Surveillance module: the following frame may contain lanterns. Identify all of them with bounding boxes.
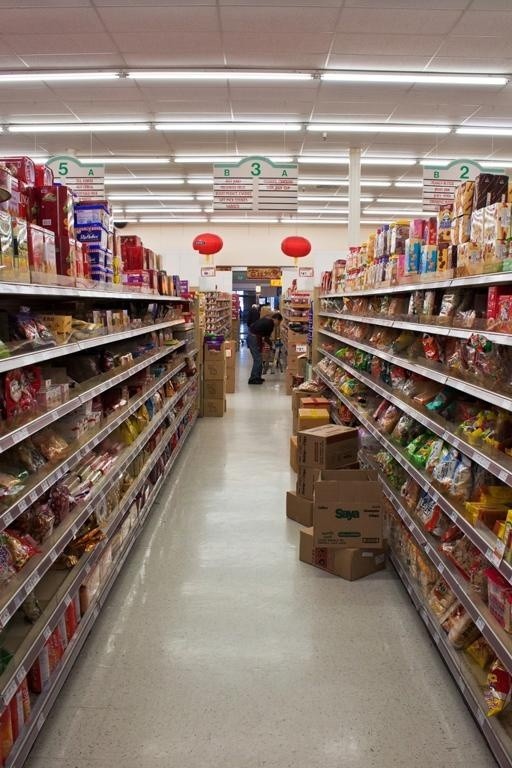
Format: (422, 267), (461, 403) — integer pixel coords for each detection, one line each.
(281, 236), (311, 271)
(192, 233), (223, 262)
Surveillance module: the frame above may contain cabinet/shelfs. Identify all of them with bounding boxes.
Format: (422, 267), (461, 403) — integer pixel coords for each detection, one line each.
(0, 283), (200, 768)
(317, 272), (512, 766)
(279, 297), (310, 375)
(199, 291), (240, 351)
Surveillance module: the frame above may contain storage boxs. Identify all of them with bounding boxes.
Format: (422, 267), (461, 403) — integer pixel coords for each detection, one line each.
(287, 328), (308, 396)
(203, 341), (235, 417)
(287, 387), (393, 581)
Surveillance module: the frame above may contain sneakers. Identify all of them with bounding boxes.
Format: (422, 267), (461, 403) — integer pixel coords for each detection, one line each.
(247, 377), (265, 385)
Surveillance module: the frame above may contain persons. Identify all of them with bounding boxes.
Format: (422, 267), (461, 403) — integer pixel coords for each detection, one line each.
(239, 304), (260, 329)
(247, 312), (283, 385)
